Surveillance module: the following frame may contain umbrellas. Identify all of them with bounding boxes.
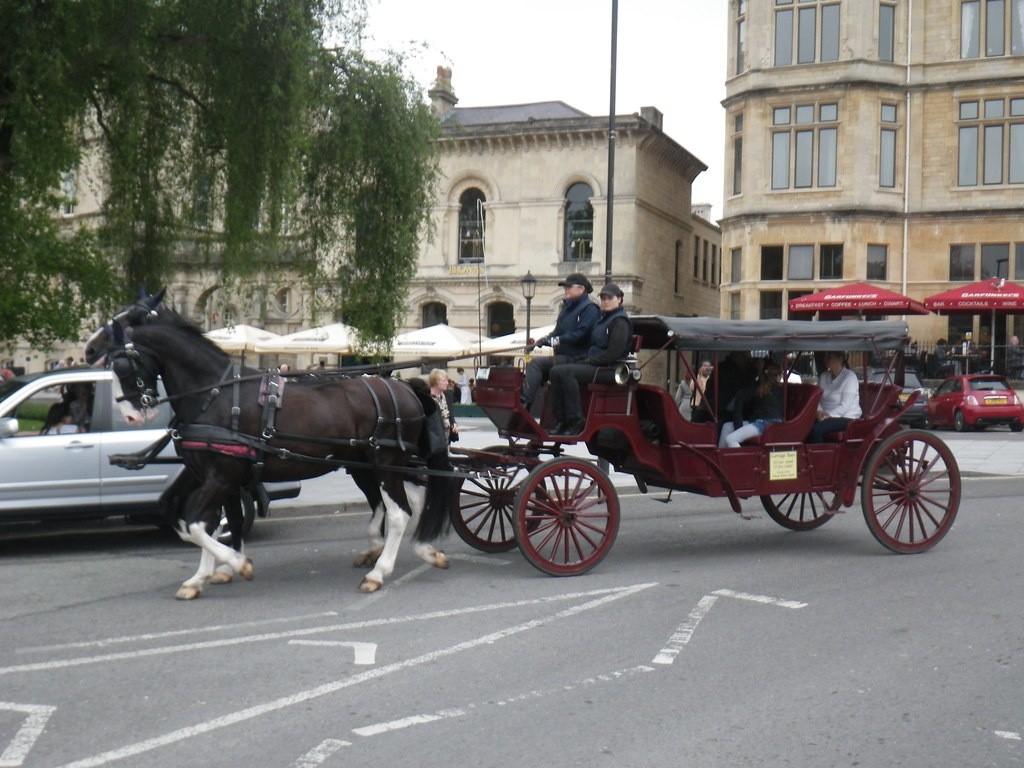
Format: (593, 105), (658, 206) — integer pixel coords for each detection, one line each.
(924, 275), (1024, 376)
(199, 320), (558, 367)
(791, 284), (929, 320)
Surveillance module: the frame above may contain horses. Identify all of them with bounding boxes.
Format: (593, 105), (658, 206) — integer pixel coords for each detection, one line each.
(86, 286), (449, 599)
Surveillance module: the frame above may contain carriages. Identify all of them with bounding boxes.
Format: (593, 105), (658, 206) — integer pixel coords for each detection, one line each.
(83, 284), (962, 600)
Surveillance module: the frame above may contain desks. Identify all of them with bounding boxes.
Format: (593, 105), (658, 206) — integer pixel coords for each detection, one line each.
(951, 354), (978, 375)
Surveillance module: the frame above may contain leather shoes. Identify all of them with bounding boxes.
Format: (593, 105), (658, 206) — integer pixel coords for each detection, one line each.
(549, 417), (587, 436)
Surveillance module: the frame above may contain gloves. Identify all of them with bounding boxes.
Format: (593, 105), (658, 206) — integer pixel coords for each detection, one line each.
(536, 337), (551, 348)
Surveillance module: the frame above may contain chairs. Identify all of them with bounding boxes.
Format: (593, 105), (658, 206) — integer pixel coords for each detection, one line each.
(921, 350), (929, 364)
(926, 354), (948, 379)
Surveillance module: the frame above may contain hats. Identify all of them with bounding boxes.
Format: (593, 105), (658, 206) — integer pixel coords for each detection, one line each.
(597, 284), (624, 298)
(558, 273), (593, 293)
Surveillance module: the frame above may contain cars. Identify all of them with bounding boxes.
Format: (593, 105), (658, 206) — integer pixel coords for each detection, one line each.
(0, 366), (302, 546)
(923, 374), (1024, 433)
(847, 365), (929, 428)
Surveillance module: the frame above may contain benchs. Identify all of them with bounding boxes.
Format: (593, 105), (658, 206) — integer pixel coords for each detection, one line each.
(825, 382), (903, 442)
(637, 384), (717, 447)
(746, 380), (825, 446)
(585, 334), (644, 415)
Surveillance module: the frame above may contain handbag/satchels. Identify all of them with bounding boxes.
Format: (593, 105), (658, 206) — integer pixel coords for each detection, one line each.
(449, 429), (459, 442)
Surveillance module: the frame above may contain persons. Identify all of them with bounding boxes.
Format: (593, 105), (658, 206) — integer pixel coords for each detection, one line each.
(457, 367), (472, 404)
(447, 380), (456, 389)
(690, 349), (862, 450)
(53, 357), (73, 370)
(469, 379), (474, 392)
(520, 273), (600, 413)
(675, 365), (695, 422)
(549, 284), (633, 436)
(428, 368), (459, 444)
(905, 336), (1024, 380)
(691, 361), (713, 422)
(41, 403), (85, 434)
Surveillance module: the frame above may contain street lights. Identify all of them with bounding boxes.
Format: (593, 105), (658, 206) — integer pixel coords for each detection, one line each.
(521, 270), (538, 371)
